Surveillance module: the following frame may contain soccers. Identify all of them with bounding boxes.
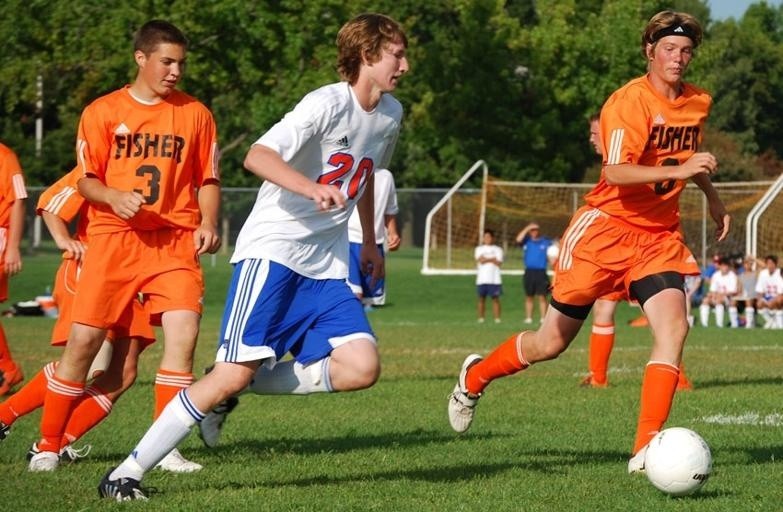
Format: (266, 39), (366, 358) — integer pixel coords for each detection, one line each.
(645, 427), (713, 500)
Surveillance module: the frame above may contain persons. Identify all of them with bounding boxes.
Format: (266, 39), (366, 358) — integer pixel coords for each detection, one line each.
(26, 20), (225, 474)
(1, 165), (158, 465)
(349, 169), (404, 307)
(474, 229), (503, 324)
(517, 223), (553, 323)
(97, 14), (410, 503)
(685, 251), (782, 329)
(447, 12), (734, 476)
(580, 114), (696, 392)
(1, 143), (30, 398)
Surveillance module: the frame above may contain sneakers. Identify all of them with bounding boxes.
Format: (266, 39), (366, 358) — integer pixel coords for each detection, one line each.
(98, 466), (156, 503)
(448, 355), (483, 433)
(154, 448), (203, 474)
(0, 364), (91, 473)
(628, 444), (648, 475)
(199, 363), (239, 450)
